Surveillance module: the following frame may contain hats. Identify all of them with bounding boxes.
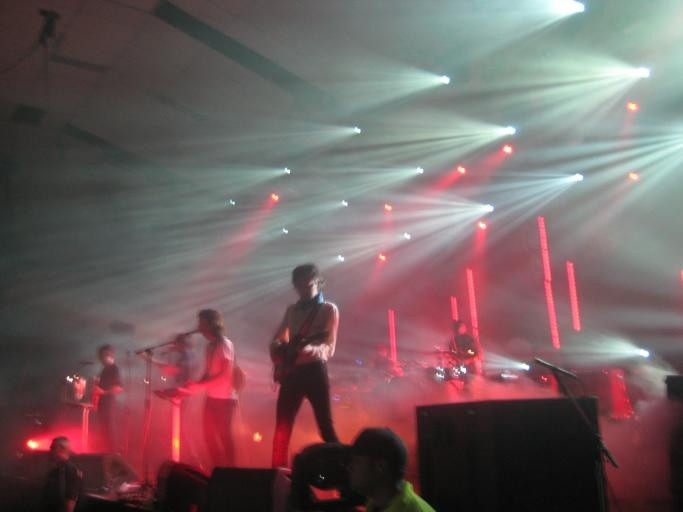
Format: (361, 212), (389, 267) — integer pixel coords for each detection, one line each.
(340, 426), (407, 466)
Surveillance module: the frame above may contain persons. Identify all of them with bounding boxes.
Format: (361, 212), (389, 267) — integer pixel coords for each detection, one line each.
(186, 307), (241, 466)
(448, 320), (481, 379)
(39, 437), (81, 512)
(269, 263), (340, 464)
(345, 426), (437, 512)
(139, 336), (201, 467)
(91, 345), (126, 456)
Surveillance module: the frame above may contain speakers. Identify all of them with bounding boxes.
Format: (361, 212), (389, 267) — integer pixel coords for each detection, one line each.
(152, 460), (208, 512)
(196, 467), (292, 512)
(69, 452), (138, 494)
(416, 396), (608, 512)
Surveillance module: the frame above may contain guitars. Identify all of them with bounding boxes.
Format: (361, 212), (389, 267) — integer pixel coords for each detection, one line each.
(151, 363), (246, 399)
(274, 329), (328, 383)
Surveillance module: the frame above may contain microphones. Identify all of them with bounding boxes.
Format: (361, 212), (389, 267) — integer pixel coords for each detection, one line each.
(534, 356), (579, 381)
(80, 362), (94, 364)
(191, 329), (199, 333)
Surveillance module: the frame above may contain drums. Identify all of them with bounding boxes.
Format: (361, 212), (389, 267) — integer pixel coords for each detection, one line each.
(447, 364), (468, 380)
(429, 364), (447, 382)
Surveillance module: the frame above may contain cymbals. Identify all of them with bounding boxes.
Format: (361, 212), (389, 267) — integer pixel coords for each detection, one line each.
(441, 350), (467, 360)
